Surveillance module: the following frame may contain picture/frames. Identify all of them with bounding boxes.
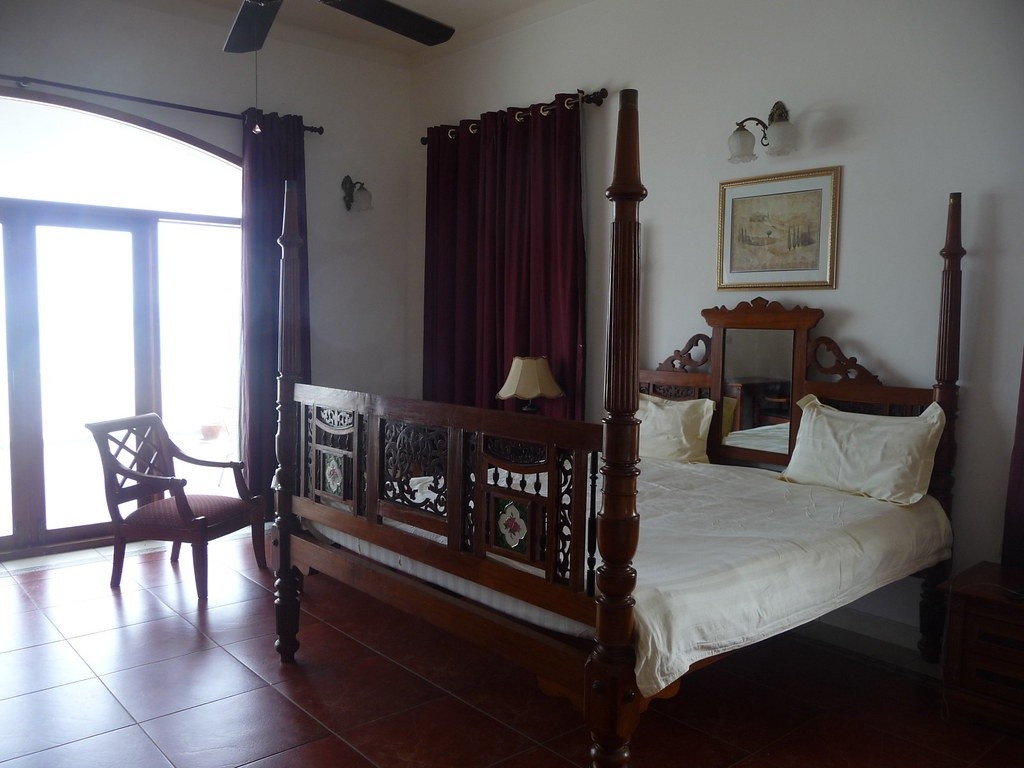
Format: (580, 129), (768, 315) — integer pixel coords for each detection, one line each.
(712, 164), (848, 292)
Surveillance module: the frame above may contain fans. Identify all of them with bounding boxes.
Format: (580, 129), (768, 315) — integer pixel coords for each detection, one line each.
(219, 0), (455, 59)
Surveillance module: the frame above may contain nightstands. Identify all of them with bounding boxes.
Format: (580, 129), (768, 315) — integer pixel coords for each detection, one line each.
(943, 559), (1023, 735)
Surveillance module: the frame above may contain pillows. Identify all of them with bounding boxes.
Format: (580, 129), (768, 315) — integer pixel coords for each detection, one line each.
(781, 392), (948, 508)
(632, 394), (715, 462)
(722, 397), (738, 443)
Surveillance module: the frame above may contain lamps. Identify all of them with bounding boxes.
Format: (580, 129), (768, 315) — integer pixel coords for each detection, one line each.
(496, 356), (565, 459)
(726, 101), (794, 166)
(337, 171), (376, 218)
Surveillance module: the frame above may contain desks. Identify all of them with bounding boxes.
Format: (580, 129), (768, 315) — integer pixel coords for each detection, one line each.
(724, 377), (790, 432)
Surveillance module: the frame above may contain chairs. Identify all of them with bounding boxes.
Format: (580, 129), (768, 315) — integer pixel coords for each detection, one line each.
(752, 394), (790, 428)
(84, 409), (268, 604)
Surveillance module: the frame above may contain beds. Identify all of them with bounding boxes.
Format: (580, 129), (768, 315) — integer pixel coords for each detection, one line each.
(271, 86), (968, 768)
(723, 422), (789, 455)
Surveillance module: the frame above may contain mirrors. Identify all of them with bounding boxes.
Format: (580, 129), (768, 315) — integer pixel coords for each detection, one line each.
(716, 321), (797, 465)
